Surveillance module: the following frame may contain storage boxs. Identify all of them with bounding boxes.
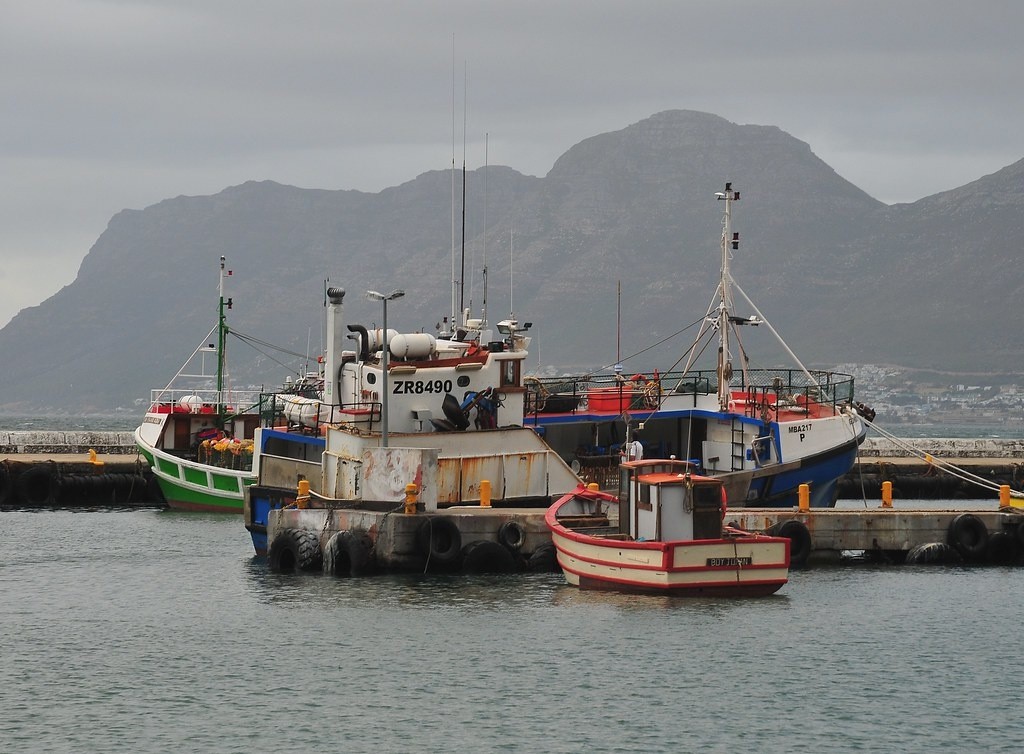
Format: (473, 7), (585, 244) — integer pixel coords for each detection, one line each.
(588, 387), (633, 411)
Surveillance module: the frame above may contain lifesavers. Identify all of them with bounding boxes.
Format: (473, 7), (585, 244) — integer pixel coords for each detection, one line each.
(947, 514), (990, 560)
(418, 516), (463, 560)
(498, 520), (526, 550)
(647, 382), (665, 395)
(772, 519), (813, 564)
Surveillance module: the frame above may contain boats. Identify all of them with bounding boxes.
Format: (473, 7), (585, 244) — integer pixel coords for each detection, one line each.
(523, 179), (875, 508)
(545, 414), (792, 593)
(134, 253), (325, 517)
(244, 289), (585, 579)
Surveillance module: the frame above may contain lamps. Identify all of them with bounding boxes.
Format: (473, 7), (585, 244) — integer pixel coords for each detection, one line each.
(455, 362), (483, 373)
(389, 365), (416, 375)
